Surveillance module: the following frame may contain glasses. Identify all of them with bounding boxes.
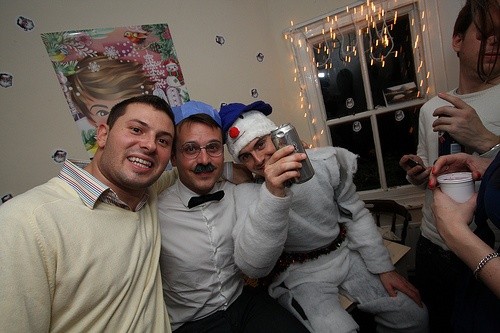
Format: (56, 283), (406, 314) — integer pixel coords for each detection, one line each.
(175, 142), (224, 159)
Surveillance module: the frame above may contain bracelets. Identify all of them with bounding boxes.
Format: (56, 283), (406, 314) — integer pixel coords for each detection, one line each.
(473, 253), (498, 274)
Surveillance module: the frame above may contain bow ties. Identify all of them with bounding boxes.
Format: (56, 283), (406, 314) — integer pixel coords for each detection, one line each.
(188, 190), (226, 209)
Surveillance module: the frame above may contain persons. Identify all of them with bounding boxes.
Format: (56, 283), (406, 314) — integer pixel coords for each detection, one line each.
(220, 101), (428, 333)
(399, 0), (500, 333)
(0, 95), (310, 333)
(156, 101), (311, 333)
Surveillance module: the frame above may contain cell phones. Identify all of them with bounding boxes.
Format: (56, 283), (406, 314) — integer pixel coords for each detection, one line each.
(407, 158), (426, 175)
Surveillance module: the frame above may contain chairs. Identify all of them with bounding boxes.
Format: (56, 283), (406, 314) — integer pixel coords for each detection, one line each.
(337, 240), (412, 309)
(361, 199), (412, 246)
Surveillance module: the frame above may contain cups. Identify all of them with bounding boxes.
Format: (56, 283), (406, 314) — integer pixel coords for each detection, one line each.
(438, 171), (476, 203)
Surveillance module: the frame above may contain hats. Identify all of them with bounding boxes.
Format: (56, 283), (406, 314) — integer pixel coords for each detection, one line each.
(218, 99), (278, 163)
(172, 100), (222, 129)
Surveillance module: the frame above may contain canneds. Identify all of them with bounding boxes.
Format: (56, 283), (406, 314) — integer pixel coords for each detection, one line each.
(438, 106), (465, 157)
(270, 123), (313, 183)
